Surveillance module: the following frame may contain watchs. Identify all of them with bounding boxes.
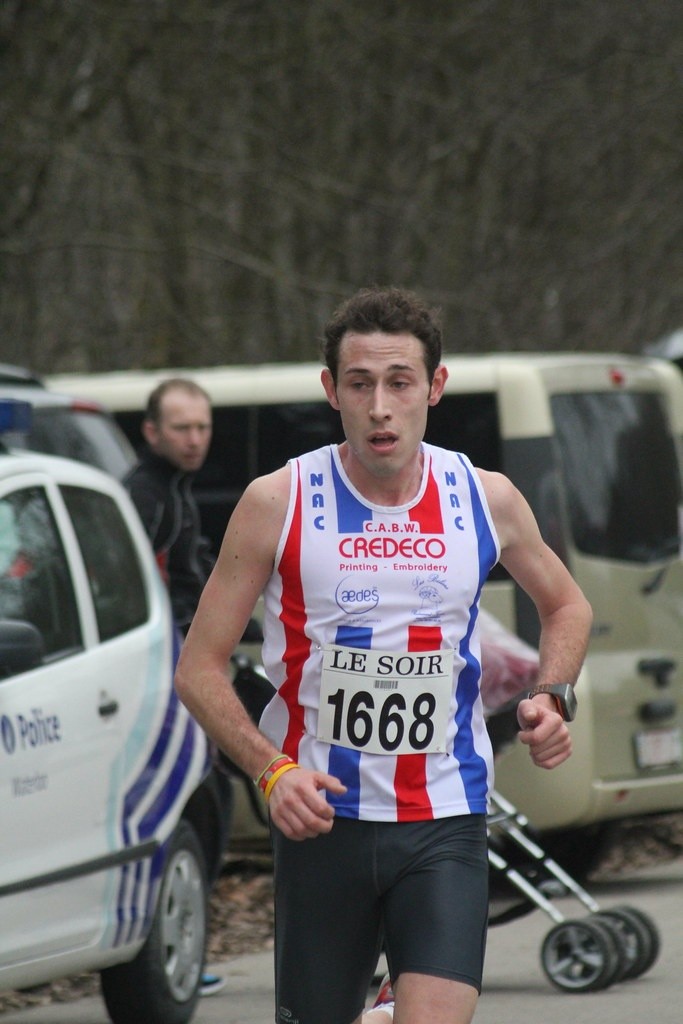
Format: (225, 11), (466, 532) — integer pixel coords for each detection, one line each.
(526, 683), (578, 722)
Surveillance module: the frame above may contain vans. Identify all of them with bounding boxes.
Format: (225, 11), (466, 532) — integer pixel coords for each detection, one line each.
(0, 353), (683, 894)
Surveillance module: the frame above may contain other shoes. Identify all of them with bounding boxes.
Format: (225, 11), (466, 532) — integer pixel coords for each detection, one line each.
(372, 973), (396, 1010)
(196, 976), (226, 996)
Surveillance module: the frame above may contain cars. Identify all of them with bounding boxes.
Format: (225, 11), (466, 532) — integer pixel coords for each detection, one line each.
(0, 453), (230, 1023)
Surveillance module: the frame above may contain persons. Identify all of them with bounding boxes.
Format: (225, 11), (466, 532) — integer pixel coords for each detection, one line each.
(120, 379), (276, 999)
(171, 287), (595, 1024)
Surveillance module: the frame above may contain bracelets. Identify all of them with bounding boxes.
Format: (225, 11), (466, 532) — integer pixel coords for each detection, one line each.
(254, 755), (301, 803)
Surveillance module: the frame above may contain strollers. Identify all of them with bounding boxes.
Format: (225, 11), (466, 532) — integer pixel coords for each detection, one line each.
(221, 621), (664, 996)
